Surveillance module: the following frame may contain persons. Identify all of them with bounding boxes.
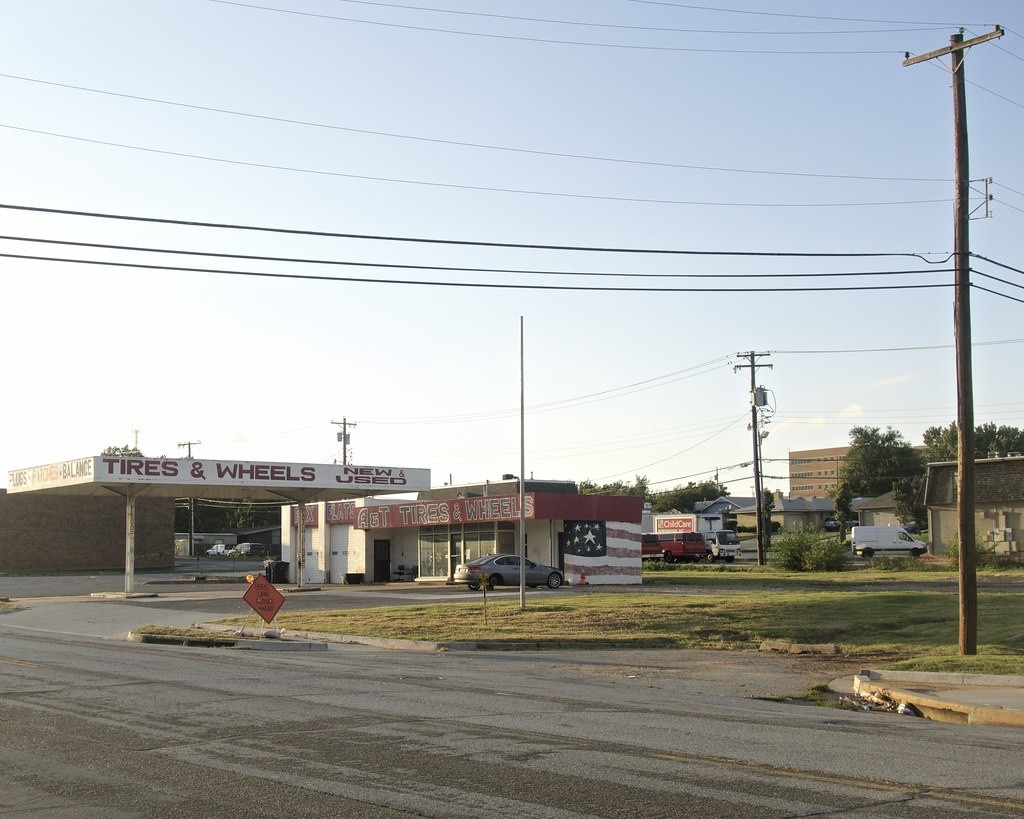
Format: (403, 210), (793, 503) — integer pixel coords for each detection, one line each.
(205, 546), (209, 558)
(897, 516), (905, 526)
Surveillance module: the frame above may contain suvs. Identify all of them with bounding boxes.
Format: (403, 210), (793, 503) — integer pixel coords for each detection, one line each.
(824, 516), (859, 532)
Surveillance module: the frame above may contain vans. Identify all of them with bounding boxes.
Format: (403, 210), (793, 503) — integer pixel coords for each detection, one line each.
(235, 543), (266, 556)
(850, 525), (928, 557)
(652, 533), (706, 563)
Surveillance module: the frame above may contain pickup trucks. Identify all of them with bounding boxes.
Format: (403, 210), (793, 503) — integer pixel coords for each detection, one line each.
(206, 543), (234, 558)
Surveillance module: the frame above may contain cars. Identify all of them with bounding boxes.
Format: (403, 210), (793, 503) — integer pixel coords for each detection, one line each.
(454, 552), (564, 591)
(903, 525), (921, 534)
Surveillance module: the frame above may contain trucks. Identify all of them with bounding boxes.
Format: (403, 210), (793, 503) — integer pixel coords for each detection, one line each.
(652, 514), (742, 563)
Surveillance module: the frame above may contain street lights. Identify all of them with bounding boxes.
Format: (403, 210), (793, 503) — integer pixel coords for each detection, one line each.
(757, 430), (770, 565)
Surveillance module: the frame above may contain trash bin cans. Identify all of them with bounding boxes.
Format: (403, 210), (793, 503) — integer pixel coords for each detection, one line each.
(264, 561), (271, 583)
(271, 561), (290, 583)
